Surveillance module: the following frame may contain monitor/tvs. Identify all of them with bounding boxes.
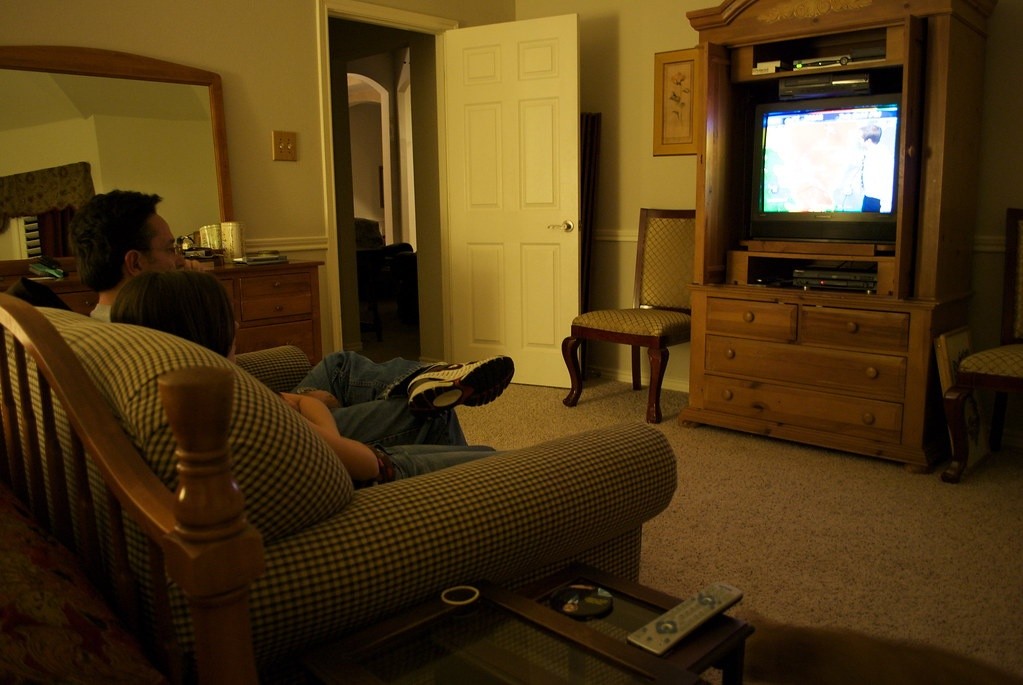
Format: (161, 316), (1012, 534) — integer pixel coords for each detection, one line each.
(749, 93), (903, 241)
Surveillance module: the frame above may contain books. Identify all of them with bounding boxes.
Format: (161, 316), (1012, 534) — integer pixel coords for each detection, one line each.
(233, 255), (289, 265)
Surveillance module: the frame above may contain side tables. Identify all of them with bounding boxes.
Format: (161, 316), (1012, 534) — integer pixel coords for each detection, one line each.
(301, 559), (755, 685)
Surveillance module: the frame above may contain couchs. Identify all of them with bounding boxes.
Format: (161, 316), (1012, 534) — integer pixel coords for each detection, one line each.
(0, 294), (679, 685)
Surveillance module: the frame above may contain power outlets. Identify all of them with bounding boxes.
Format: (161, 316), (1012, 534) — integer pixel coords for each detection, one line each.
(274, 130), (298, 162)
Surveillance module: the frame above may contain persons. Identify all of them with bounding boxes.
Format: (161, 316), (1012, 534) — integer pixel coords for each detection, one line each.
(859, 124), (881, 190)
(111, 271), (506, 485)
(68, 190), (514, 443)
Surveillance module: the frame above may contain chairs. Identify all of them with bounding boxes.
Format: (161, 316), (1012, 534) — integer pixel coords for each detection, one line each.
(562, 208), (695, 424)
(940, 205), (1023, 484)
(352, 218), (412, 342)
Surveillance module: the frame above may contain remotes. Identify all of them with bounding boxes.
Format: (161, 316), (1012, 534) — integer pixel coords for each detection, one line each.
(626, 582), (747, 657)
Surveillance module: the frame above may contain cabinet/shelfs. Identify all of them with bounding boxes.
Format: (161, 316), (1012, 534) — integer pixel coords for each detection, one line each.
(1, 255), (320, 387)
(678, 0), (998, 473)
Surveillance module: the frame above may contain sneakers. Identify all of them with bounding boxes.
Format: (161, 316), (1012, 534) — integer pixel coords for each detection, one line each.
(406, 355), (515, 417)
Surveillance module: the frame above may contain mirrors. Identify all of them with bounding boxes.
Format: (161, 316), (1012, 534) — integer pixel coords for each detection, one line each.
(0, 45), (233, 262)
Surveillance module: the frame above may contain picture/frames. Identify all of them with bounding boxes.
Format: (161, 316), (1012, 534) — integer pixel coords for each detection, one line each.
(652, 47), (700, 156)
(932, 326), (989, 469)
